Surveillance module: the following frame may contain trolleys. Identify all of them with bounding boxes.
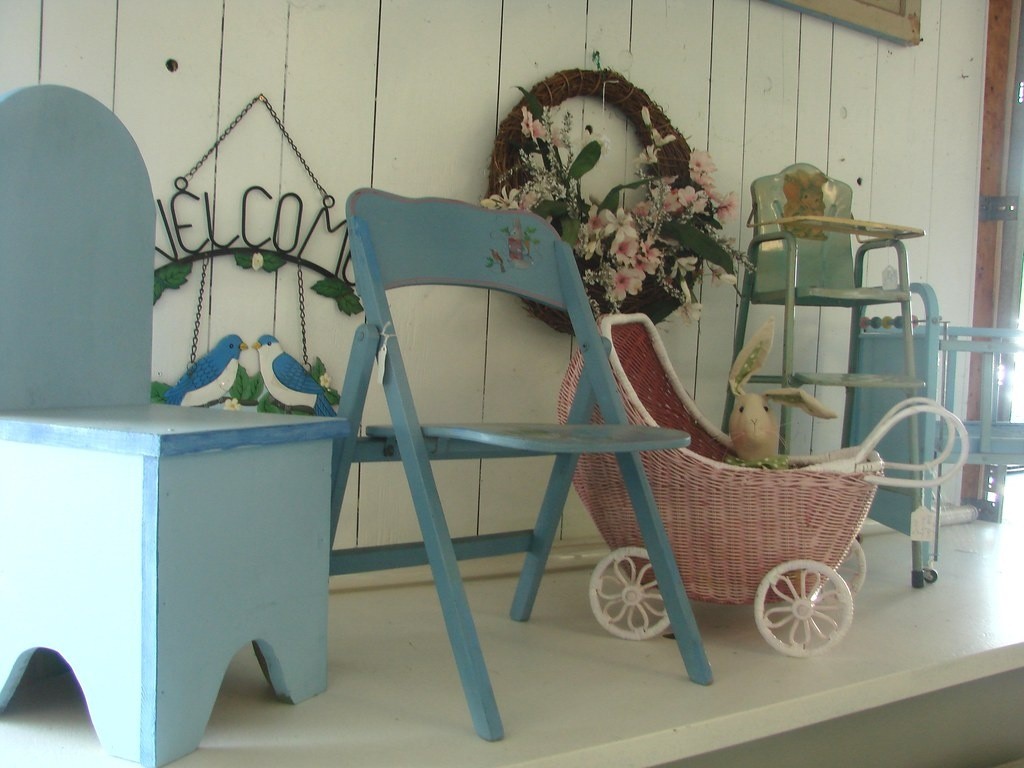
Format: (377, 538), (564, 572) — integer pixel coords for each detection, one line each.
(560, 316), (970, 658)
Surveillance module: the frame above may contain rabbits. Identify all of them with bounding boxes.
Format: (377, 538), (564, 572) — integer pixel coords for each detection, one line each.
(721, 317), (839, 470)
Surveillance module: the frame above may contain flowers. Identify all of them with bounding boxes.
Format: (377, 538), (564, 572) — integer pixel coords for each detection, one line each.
(481, 61), (753, 328)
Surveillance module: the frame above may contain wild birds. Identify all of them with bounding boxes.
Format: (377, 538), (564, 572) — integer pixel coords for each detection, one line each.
(162, 333), (251, 408)
(252, 333), (338, 420)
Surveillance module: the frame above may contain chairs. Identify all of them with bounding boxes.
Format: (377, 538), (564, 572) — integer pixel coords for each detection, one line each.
(847, 275), (1023, 581)
(1, 84), (340, 766)
(720, 164), (938, 584)
(298, 184), (716, 740)
